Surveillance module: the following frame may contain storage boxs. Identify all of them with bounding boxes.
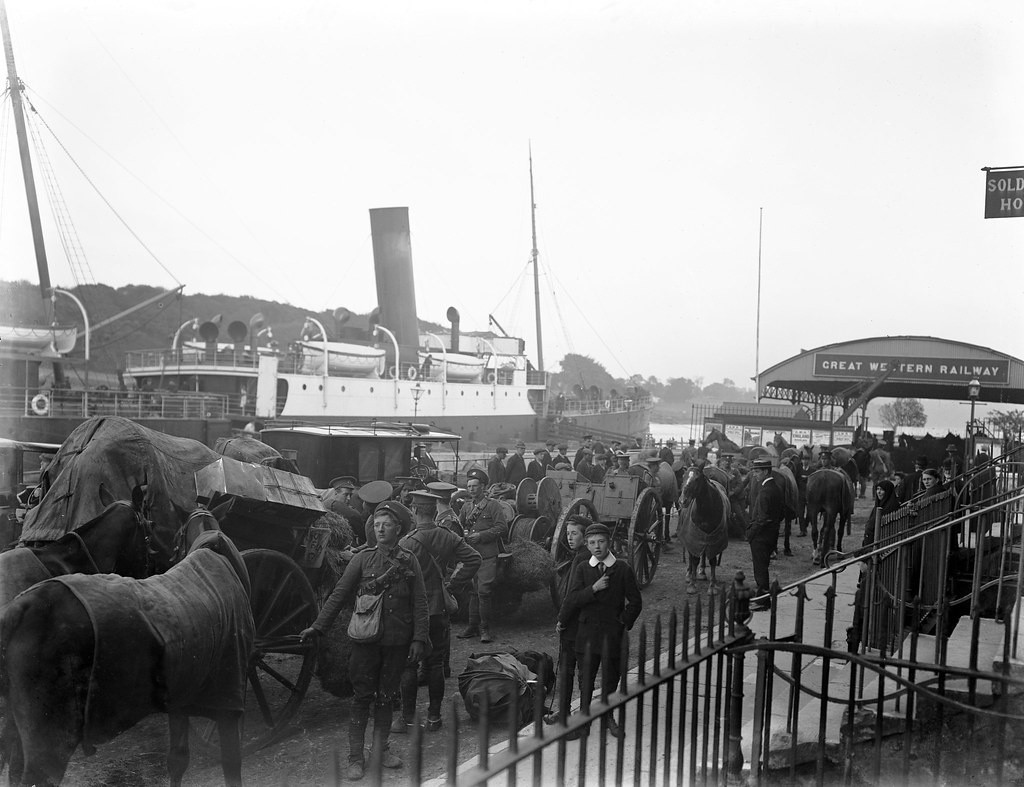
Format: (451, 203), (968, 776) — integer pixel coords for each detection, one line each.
(546, 470), (651, 521)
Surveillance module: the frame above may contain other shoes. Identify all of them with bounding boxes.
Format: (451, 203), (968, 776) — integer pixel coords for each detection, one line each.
(797, 531), (806, 537)
(750, 599), (772, 611)
(347, 755), (365, 780)
(390, 717), (412, 732)
(369, 745), (403, 768)
(480, 624), (492, 641)
(426, 715), (443, 731)
(609, 718), (624, 738)
(456, 625), (480, 638)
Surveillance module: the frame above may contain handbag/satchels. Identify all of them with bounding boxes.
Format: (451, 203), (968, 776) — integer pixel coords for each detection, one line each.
(346, 594), (384, 643)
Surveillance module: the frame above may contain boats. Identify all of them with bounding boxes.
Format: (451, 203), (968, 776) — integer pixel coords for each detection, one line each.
(1, 209), (652, 458)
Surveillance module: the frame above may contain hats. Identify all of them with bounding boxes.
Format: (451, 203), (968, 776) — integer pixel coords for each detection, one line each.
(819, 451), (832, 458)
(584, 524), (610, 534)
(358, 481), (393, 504)
(375, 500), (411, 536)
(329, 476), (357, 489)
(749, 459), (776, 469)
(394, 476), (421, 486)
(721, 455), (734, 461)
(912, 454), (928, 466)
(569, 515), (592, 525)
(495, 435), (630, 469)
(946, 444), (957, 451)
(409, 491), (443, 504)
(427, 482), (457, 495)
(467, 469), (489, 484)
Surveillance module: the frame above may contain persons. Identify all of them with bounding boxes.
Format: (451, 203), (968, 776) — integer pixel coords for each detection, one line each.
(57, 378), (248, 418)
(563, 524), (641, 740)
(527, 361), (535, 385)
(391, 490), (483, 733)
(21, 452), (54, 510)
(299, 501), (429, 780)
(421, 355), (433, 382)
(329, 436), (996, 637)
(544, 514), (604, 723)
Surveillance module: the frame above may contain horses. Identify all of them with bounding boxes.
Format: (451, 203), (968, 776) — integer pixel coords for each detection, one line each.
(701, 424), (897, 569)
(0, 482), (155, 609)
(0, 496), (256, 787)
(676, 462), (731, 596)
